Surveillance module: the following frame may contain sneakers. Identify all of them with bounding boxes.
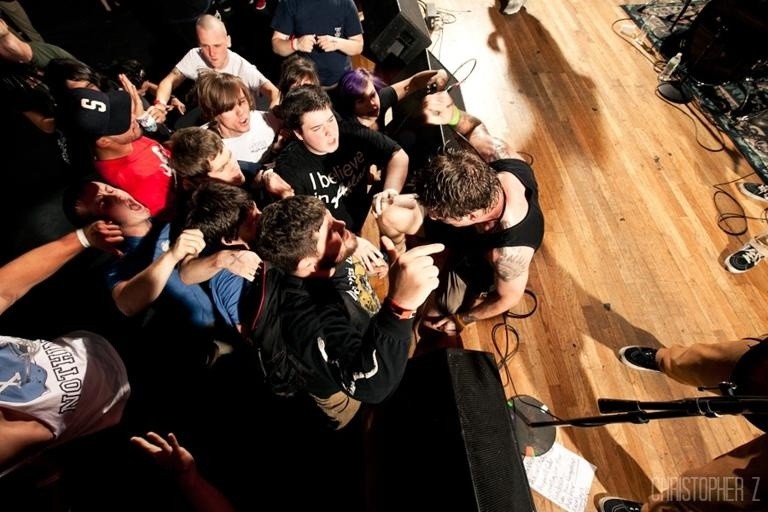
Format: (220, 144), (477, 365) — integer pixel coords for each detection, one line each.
(599, 494), (643, 512)
(739, 181), (767, 201)
(618, 344), (661, 373)
(724, 240), (761, 274)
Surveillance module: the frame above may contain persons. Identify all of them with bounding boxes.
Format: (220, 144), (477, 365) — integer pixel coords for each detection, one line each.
(723, 179), (768, 276)
(593, 332), (768, 511)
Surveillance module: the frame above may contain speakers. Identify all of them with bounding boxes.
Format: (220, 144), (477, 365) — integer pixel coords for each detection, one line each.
(361, 0), (432, 72)
(391, 49), (471, 181)
(380, 347), (535, 512)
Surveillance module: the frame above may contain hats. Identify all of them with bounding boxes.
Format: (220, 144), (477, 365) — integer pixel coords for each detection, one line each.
(54, 88), (130, 141)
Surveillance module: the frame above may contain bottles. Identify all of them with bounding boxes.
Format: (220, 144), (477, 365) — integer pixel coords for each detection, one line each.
(634, 13), (655, 45)
(658, 53), (682, 81)
